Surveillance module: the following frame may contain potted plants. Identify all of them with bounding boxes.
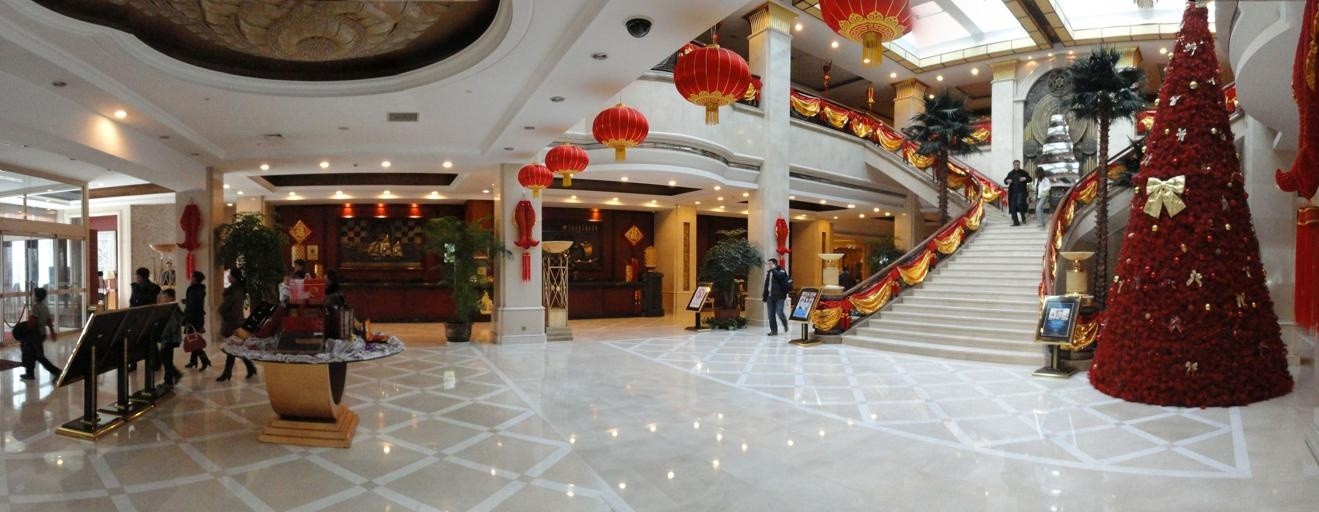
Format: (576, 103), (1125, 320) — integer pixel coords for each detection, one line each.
(727, 318), (737, 330)
(737, 316), (747, 329)
(220, 211), (292, 318)
(712, 320), (720, 329)
(696, 227), (766, 328)
(423, 214), (514, 342)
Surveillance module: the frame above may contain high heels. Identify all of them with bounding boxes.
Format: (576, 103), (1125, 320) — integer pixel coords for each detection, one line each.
(184, 350), (199, 370)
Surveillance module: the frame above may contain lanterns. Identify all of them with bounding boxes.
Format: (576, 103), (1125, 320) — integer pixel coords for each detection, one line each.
(673, 42), (752, 127)
(592, 102), (650, 161)
(819, 0), (913, 68)
(544, 142), (589, 188)
(517, 163), (553, 198)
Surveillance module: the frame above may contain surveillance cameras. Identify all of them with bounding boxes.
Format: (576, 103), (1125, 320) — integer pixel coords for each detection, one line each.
(623, 17), (653, 38)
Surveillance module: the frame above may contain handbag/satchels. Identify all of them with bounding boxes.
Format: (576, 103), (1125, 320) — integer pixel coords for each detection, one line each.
(182, 323), (207, 353)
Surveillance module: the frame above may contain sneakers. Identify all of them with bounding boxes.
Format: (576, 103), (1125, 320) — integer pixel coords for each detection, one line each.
(174, 372), (184, 385)
(156, 382), (174, 390)
(767, 331), (778, 336)
(18, 373), (35, 381)
(784, 325), (789, 333)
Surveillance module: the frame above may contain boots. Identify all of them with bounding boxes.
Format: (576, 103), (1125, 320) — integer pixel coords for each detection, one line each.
(199, 350), (213, 372)
(241, 358), (257, 379)
(216, 352), (236, 382)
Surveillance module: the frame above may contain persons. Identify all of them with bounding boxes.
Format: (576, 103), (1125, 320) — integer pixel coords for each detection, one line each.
(1034, 167), (1051, 229)
(19, 287), (62, 384)
(126, 258), (344, 386)
(761, 258), (790, 336)
(1003, 160), (1032, 227)
(839, 265), (851, 291)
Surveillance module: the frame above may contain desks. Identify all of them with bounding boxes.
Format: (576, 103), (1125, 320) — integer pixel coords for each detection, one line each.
(219, 330), (406, 448)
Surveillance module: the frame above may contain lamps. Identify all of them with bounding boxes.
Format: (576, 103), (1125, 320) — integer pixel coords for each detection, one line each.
(540, 239), (575, 341)
(816, 252), (845, 285)
(1058, 252), (1095, 296)
(339, 203), (424, 219)
(149, 243), (175, 303)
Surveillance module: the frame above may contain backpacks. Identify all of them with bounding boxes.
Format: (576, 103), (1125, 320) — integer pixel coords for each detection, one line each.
(773, 267), (794, 294)
